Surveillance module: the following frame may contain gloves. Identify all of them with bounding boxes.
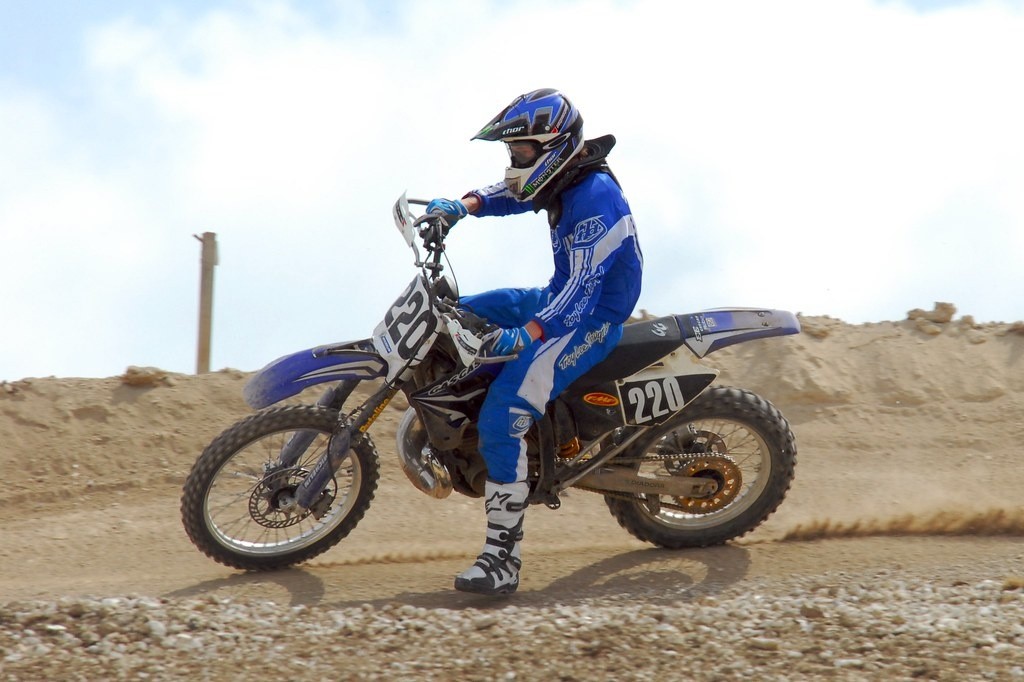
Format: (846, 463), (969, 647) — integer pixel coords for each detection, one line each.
(490, 327), (533, 357)
(427, 195), (468, 237)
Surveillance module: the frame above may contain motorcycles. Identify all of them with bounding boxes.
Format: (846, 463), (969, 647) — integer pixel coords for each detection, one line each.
(178, 192), (801, 571)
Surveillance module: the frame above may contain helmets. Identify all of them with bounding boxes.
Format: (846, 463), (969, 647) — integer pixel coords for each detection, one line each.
(467, 88), (586, 202)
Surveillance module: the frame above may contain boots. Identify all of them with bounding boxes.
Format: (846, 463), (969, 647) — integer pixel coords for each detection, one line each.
(453, 479), (531, 594)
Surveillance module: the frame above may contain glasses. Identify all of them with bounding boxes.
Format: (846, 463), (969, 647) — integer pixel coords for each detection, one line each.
(505, 137), (543, 168)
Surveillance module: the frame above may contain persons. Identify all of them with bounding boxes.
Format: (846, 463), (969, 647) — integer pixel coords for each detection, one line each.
(413, 89), (644, 595)
